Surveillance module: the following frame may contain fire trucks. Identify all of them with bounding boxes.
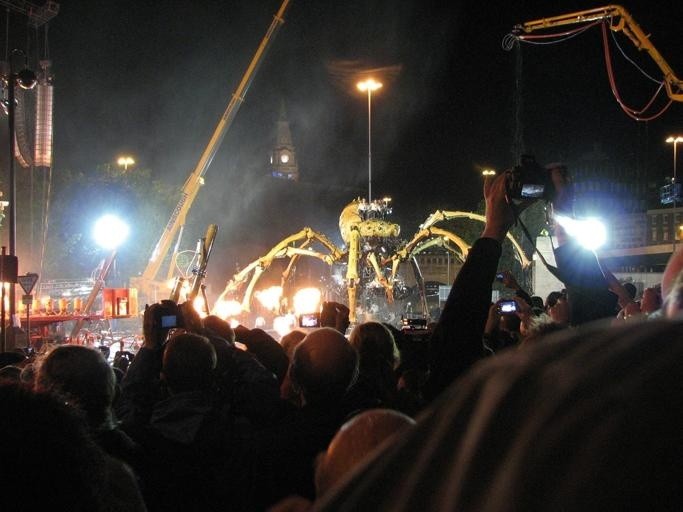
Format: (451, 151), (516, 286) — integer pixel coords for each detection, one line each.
(1, 249), (119, 355)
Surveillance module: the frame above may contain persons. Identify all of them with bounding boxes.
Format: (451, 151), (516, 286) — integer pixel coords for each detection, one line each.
(1, 162), (682, 511)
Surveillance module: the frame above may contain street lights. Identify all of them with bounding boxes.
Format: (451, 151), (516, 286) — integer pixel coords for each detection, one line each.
(665, 135), (682, 254)
(356, 76), (378, 204)
(483, 168), (496, 188)
(117, 155), (134, 208)
(7, 69), (39, 331)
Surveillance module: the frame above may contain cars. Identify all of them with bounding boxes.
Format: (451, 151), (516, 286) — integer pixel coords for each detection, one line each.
(386, 309), (428, 335)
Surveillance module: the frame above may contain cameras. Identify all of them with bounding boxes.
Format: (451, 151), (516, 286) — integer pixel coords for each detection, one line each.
(299, 312), (323, 328)
(496, 272), (505, 281)
(508, 164), (554, 198)
(497, 299), (518, 315)
(157, 302), (180, 327)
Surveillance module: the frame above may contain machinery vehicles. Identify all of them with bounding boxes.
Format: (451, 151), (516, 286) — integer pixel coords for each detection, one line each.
(122, 0), (294, 316)
